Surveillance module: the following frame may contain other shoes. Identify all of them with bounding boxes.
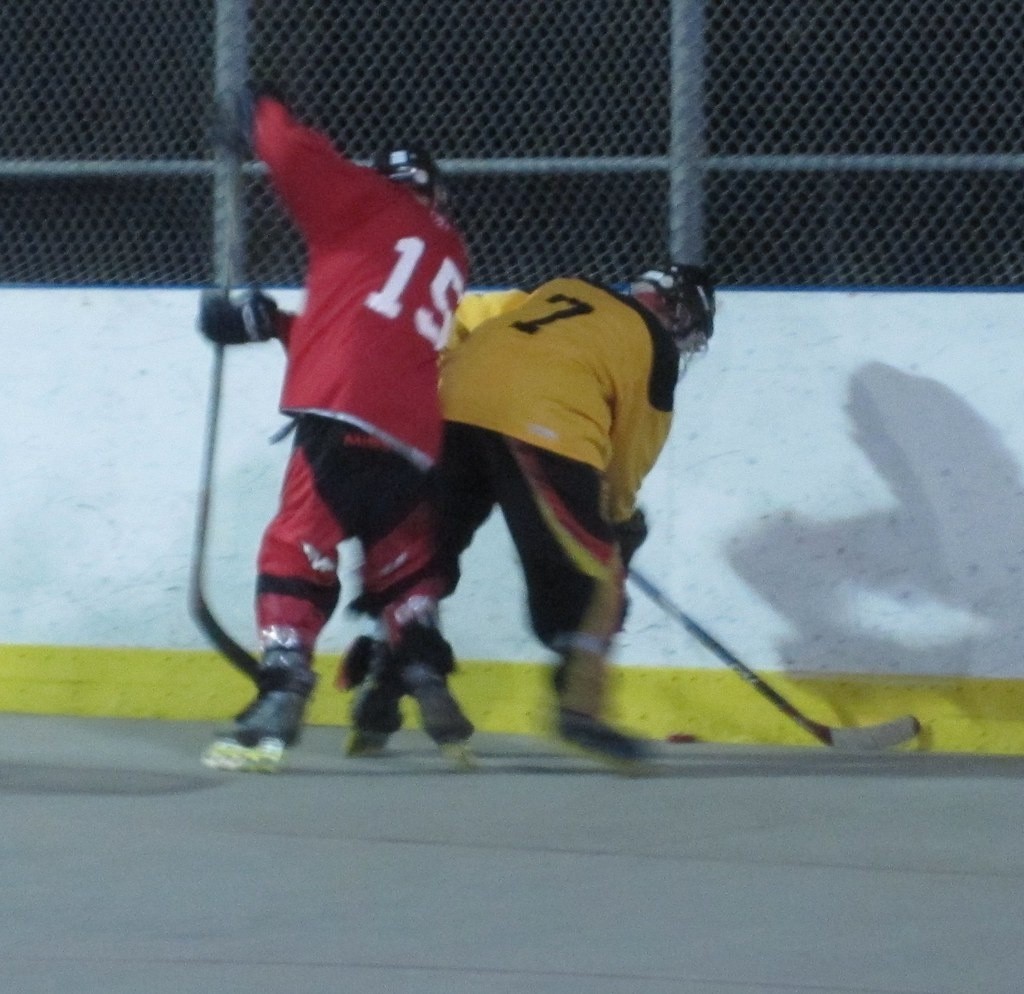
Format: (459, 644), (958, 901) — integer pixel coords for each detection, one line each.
(551, 705), (639, 776)
(199, 648), (318, 773)
(346, 688), (402, 757)
(413, 682), (474, 758)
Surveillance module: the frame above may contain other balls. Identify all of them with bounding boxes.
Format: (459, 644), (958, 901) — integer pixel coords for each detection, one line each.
(665, 733), (694, 744)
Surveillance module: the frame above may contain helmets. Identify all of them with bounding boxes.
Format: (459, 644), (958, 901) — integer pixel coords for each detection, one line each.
(372, 145), (435, 198)
(636, 262), (715, 339)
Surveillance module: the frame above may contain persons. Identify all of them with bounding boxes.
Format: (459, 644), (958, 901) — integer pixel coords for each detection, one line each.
(335, 265), (716, 771)
(199, 81), (475, 771)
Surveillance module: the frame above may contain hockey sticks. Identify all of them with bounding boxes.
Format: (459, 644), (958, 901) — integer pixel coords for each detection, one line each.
(185, 126), (282, 689)
(624, 563), (921, 752)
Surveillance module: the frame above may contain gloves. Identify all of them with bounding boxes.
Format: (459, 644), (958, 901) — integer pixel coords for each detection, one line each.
(618, 510), (647, 566)
(200, 287), (278, 344)
